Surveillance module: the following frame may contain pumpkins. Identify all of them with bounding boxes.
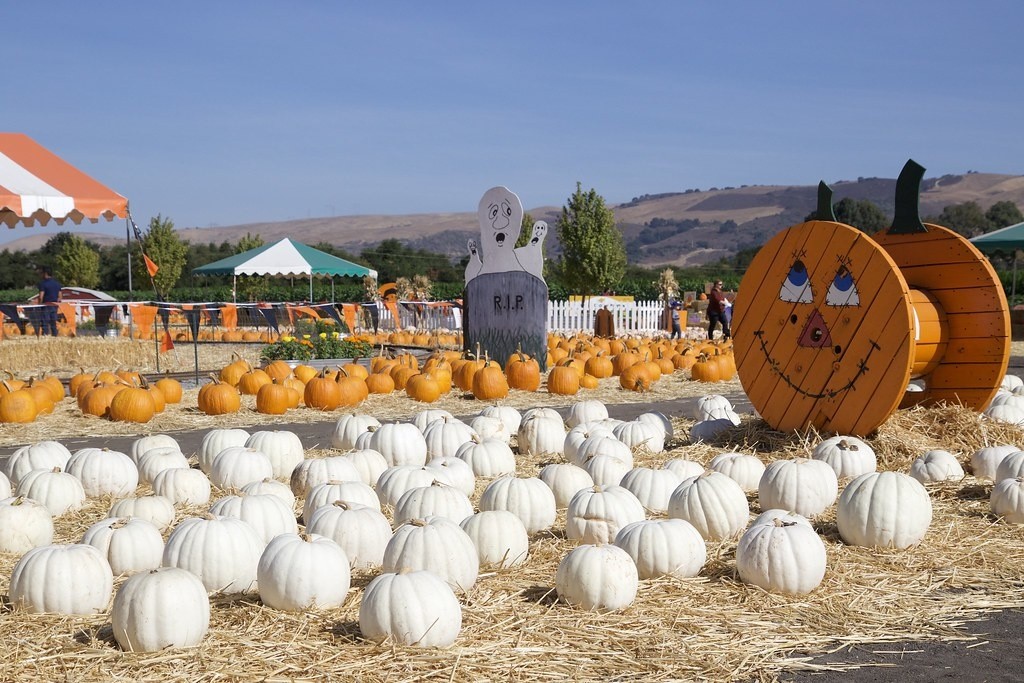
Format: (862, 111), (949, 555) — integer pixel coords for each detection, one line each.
(0, 402), (564, 656)
(0, 343), (541, 423)
(354, 328), (465, 346)
(3, 320), (72, 338)
(119, 327), (291, 343)
(547, 374), (1024, 613)
(545, 329), (737, 395)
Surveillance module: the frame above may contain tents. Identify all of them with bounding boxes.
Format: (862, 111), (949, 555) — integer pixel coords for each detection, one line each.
(0, 130), (133, 304)
(191, 238), (379, 326)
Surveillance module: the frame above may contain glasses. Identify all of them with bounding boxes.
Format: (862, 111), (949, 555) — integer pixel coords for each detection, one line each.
(717, 284), (722, 286)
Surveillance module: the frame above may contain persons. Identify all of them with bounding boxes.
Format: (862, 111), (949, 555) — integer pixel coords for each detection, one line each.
(671, 302), (681, 339)
(40, 268), (62, 337)
(707, 279), (731, 342)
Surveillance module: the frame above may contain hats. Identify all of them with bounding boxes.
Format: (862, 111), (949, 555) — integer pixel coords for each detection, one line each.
(671, 300), (682, 306)
